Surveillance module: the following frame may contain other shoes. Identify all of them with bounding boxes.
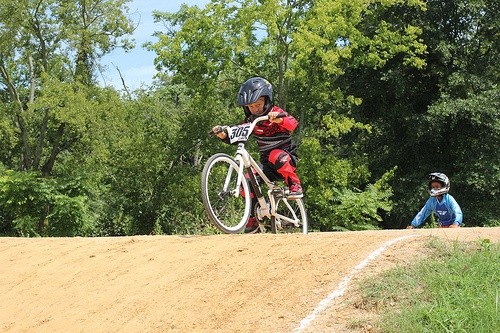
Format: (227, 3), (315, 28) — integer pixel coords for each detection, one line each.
(287, 192), (304, 200)
(245, 217), (259, 234)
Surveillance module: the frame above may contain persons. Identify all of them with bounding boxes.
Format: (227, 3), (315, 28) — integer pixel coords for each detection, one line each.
(406, 173), (463, 229)
(212, 77), (303, 233)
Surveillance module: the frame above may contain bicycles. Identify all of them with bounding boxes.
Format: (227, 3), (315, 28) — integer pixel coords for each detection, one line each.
(201, 111), (308, 234)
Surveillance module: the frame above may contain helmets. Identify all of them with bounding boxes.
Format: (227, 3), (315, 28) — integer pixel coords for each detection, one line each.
(428, 172), (451, 196)
(238, 76), (273, 116)
